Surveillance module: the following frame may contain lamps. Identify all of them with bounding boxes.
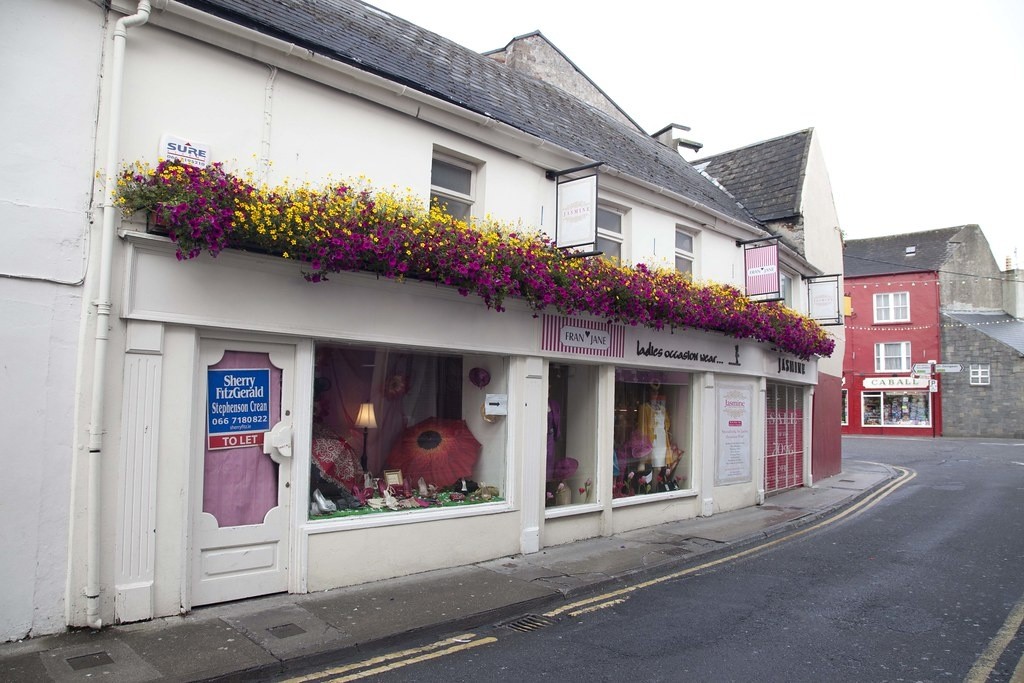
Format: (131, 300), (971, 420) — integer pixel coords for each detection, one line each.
(354, 401), (379, 472)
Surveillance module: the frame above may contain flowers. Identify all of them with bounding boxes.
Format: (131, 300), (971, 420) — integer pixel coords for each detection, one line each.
(113, 150), (841, 360)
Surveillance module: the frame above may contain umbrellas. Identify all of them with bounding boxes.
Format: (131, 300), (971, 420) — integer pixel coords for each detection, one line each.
(387, 416), (481, 492)
(311, 422), (365, 508)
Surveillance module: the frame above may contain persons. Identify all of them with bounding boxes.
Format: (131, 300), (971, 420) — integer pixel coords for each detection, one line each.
(546, 384), (560, 498)
(637, 389), (671, 488)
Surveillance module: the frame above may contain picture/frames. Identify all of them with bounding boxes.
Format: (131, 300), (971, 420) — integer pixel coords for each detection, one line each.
(383, 469), (404, 485)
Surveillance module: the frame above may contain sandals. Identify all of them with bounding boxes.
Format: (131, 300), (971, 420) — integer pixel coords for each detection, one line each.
(312, 471), (464, 515)
